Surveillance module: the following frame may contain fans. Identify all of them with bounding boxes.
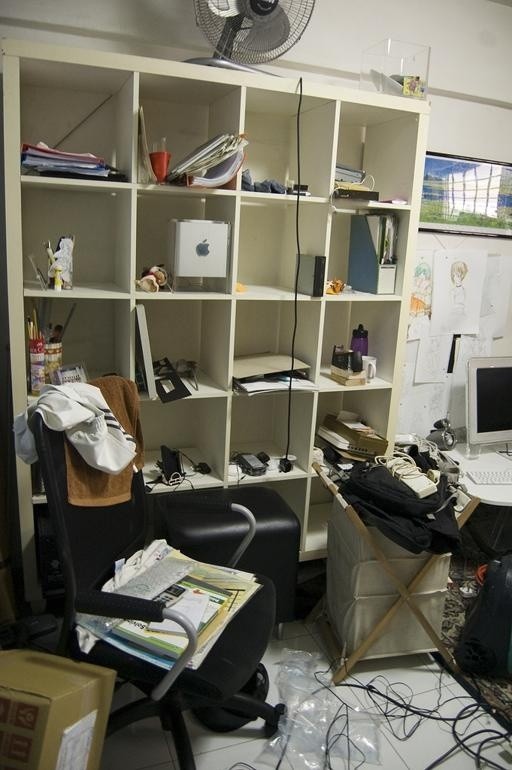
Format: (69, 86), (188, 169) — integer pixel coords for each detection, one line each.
(183, 0), (317, 77)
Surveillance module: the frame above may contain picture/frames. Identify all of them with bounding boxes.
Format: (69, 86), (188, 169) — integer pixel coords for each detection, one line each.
(418, 151), (512, 238)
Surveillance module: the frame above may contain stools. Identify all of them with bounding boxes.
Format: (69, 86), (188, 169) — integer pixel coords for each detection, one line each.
(158, 483), (301, 640)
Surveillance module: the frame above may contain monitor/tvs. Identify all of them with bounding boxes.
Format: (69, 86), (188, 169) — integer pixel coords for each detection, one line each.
(466, 356), (512, 460)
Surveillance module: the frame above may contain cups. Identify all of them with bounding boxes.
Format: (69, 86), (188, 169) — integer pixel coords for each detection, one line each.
(361, 356), (377, 380)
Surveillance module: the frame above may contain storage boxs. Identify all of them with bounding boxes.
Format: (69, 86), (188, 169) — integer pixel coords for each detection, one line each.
(0, 649), (118, 770)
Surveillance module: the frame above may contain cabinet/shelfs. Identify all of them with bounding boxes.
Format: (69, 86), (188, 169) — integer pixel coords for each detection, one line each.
(0, 36), (430, 616)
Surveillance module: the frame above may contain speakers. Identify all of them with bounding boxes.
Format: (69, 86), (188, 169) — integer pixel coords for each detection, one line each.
(426, 419), (456, 450)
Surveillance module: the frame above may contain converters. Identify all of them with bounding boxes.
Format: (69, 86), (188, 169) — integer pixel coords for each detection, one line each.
(239, 453), (265, 477)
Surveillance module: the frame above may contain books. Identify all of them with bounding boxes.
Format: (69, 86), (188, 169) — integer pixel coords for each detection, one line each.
(16, 142), (121, 181)
(79, 540), (266, 677)
(166, 129), (249, 190)
(317, 408), (388, 470)
(230, 351), (313, 399)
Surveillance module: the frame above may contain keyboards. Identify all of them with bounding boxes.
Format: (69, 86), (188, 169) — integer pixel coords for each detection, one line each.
(466, 470), (512, 484)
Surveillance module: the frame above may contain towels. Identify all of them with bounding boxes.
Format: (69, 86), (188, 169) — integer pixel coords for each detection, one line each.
(61, 378), (145, 509)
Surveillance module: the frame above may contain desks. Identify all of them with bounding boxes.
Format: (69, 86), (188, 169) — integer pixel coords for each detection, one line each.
(441, 438), (512, 564)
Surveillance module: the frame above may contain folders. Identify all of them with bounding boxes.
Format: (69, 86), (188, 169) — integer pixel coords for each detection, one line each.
(21, 142), (120, 172)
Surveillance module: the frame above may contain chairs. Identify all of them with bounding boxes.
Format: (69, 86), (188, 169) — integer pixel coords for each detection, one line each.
(27, 373), (289, 770)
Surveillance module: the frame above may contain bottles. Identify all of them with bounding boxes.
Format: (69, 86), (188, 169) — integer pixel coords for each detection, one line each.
(350, 324), (368, 355)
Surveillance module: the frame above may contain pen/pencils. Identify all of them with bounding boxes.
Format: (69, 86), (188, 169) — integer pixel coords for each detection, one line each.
(28, 297), (76, 343)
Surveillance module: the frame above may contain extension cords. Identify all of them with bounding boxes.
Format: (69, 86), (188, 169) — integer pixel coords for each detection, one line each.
(385, 457), (438, 499)
